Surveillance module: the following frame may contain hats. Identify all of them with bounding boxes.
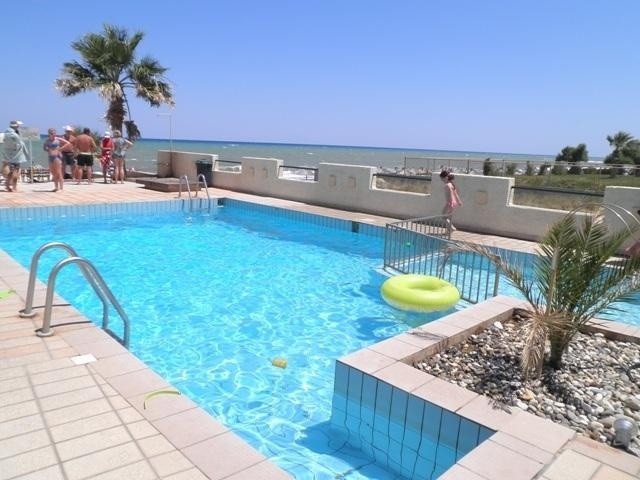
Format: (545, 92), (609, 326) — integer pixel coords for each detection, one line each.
(64, 126), (73, 132)
(10, 120), (24, 127)
(103, 131), (110, 139)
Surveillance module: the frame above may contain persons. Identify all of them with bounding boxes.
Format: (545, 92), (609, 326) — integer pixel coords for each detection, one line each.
(61, 125), (78, 183)
(100, 131), (115, 184)
(3, 120), (30, 193)
(112, 130), (134, 184)
(43, 127), (71, 194)
(73, 128), (97, 185)
(440, 168), (463, 235)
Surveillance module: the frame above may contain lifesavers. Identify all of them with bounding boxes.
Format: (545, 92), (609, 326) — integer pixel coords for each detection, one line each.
(380, 273), (460, 313)
